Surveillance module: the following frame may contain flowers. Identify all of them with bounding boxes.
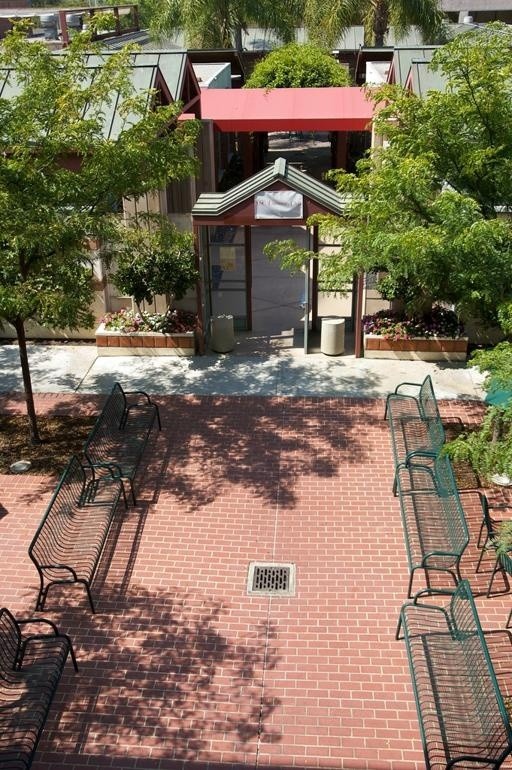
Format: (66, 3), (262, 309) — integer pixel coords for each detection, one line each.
(99, 306), (193, 335)
(361, 308), (460, 342)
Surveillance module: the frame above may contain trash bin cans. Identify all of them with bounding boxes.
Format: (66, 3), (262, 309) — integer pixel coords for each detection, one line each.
(320, 317), (345, 356)
(210, 315), (235, 354)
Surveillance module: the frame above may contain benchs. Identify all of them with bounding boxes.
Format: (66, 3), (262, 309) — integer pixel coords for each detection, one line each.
(474, 495), (510, 570)
(1, 606), (79, 768)
(28, 455), (130, 609)
(390, 450), (467, 598)
(384, 377), (448, 495)
(487, 552), (512, 629)
(83, 383), (162, 502)
(396, 583), (510, 767)
